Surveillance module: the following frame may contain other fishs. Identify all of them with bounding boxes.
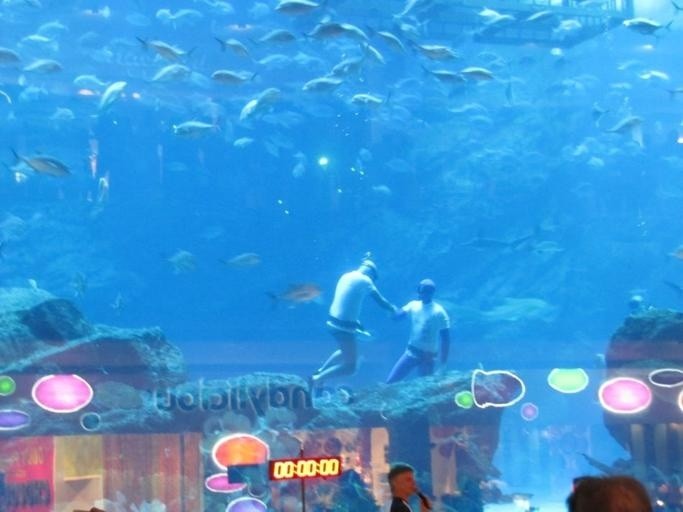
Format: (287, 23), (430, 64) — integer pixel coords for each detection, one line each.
(0, 0), (682, 198)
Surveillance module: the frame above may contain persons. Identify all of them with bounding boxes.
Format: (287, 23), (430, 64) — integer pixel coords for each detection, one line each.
(310, 259), (397, 398)
(387, 462), (432, 511)
(385, 279), (450, 385)
(565, 476), (652, 512)
